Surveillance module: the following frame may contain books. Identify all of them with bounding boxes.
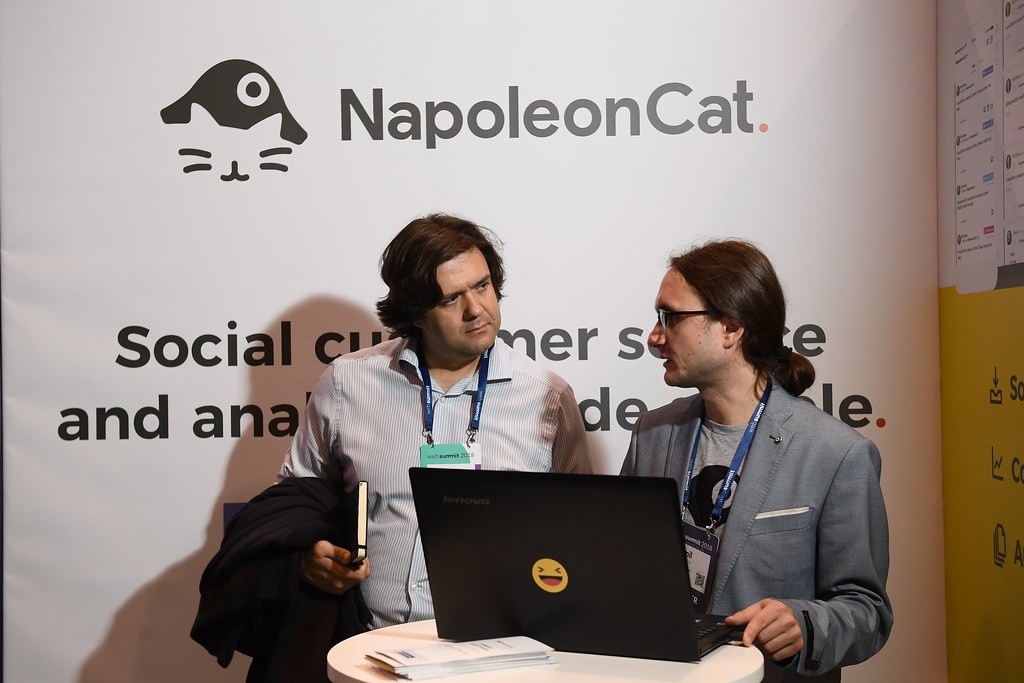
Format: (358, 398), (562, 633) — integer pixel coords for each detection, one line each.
(329, 480), (369, 566)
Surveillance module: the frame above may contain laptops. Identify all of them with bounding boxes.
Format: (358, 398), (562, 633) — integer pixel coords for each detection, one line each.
(408, 467), (739, 662)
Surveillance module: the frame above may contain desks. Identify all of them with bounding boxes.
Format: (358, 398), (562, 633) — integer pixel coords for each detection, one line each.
(327, 619), (764, 683)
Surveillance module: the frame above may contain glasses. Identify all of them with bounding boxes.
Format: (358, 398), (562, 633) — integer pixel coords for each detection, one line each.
(657, 305), (720, 331)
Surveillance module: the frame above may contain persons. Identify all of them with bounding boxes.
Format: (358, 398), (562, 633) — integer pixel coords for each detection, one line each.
(276, 213), (595, 631)
(619, 240), (894, 683)
